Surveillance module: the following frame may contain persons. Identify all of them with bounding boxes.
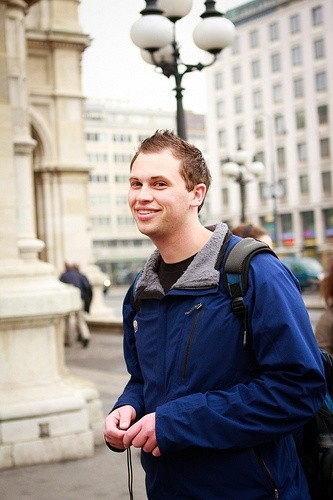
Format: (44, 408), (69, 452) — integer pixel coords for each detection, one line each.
(232, 226), (272, 256)
(104, 128), (333, 500)
(57, 261), (93, 346)
(293, 248), (333, 500)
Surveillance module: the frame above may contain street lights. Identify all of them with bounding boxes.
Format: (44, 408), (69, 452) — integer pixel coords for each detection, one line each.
(130, 1), (236, 141)
(219, 144), (265, 224)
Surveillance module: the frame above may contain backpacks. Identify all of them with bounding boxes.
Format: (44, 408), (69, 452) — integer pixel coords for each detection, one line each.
(129, 239), (333, 500)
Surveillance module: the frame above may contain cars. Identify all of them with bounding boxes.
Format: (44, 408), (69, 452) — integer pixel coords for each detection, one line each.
(285, 257), (326, 293)
